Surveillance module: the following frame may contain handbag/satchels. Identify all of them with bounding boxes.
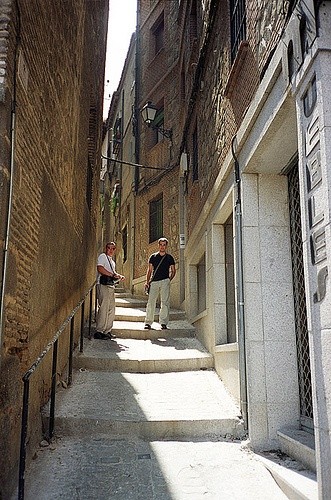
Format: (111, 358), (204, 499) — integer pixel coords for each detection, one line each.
(99, 275), (119, 285)
(144, 282), (150, 296)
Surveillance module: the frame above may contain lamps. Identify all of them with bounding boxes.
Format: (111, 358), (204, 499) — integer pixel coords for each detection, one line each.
(140, 100), (173, 138)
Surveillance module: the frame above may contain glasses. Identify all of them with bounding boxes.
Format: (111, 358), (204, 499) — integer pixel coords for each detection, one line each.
(107, 247), (116, 250)
(160, 243), (165, 246)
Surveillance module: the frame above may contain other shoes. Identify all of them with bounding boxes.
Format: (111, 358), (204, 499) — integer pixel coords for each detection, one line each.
(94, 331), (116, 340)
(161, 324), (167, 329)
(144, 324), (151, 329)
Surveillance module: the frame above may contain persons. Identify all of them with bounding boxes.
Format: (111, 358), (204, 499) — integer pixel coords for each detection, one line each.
(143, 238), (177, 331)
(94, 242), (125, 341)
